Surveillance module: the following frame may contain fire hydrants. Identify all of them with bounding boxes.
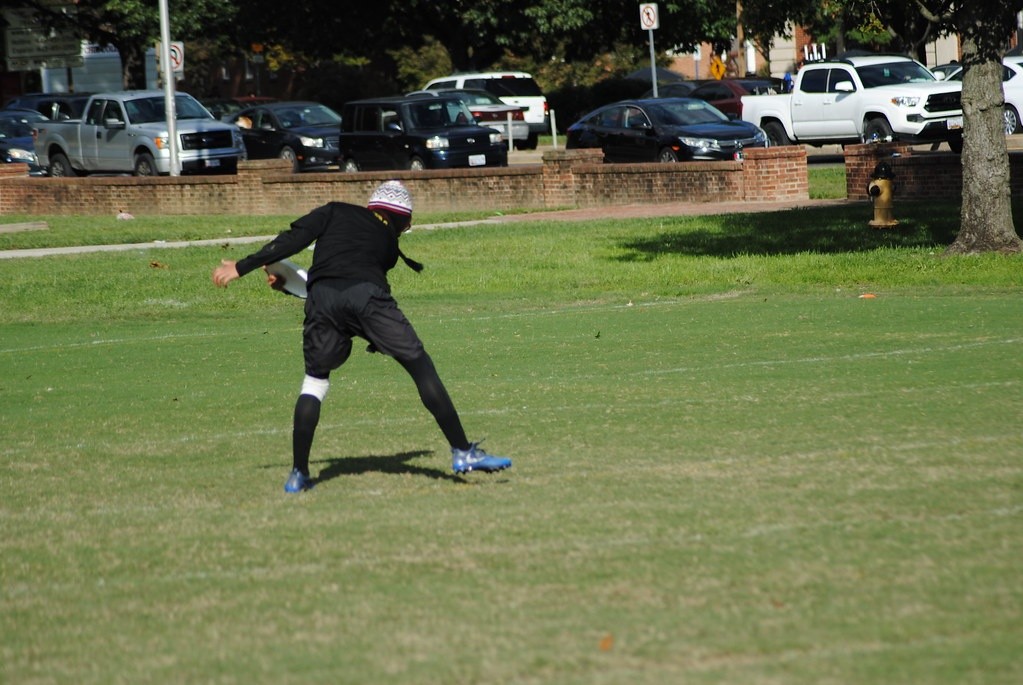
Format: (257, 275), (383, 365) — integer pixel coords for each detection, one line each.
(867, 164), (899, 229)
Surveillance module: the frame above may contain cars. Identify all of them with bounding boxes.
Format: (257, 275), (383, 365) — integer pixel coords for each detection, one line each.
(639, 78), (794, 122)
(566, 98), (771, 163)
(7, 91), (156, 120)
(0, 107), (58, 181)
(227, 101), (343, 172)
(404, 88), (528, 145)
(338, 95), (508, 172)
(150, 98), (277, 120)
(926, 55), (1023, 135)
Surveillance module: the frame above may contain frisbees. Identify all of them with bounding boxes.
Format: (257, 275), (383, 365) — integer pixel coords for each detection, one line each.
(267, 259), (310, 300)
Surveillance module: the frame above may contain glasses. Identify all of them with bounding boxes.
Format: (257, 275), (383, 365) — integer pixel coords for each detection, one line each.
(402, 222), (411, 232)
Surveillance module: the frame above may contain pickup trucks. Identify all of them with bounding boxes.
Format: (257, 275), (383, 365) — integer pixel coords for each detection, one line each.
(739, 51), (963, 154)
(31, 90), (243, 177)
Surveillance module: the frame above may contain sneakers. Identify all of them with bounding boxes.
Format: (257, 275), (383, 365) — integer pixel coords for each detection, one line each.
(285, 468), (310, 492)
(452, 439), (511, 474)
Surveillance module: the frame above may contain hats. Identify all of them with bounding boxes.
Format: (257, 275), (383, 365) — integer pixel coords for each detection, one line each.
(367, 180), (412, 220)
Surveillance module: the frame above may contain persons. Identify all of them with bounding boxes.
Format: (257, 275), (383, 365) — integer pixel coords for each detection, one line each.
(213, 179), (512, 493)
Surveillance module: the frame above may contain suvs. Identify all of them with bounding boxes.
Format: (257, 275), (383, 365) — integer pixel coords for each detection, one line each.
(422, 72), (550, 150)
